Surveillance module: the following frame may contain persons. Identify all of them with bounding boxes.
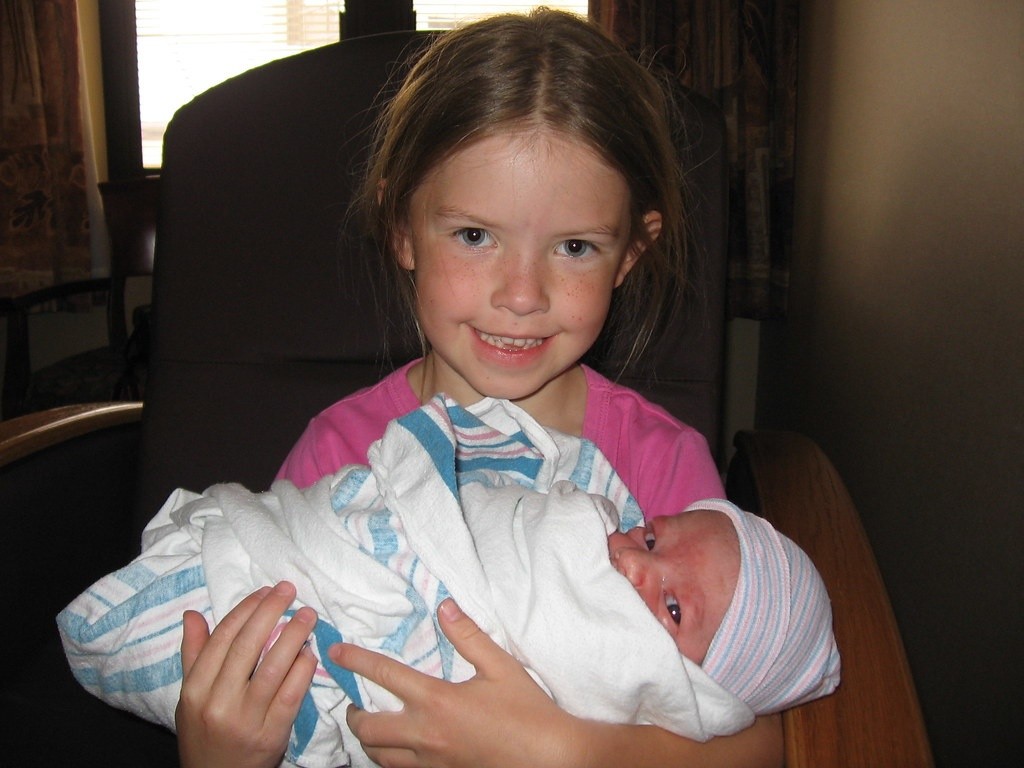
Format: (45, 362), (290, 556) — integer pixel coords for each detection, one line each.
(55, 5), (841, 768)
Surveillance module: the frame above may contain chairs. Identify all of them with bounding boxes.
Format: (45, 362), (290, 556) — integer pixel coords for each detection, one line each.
(0, 170), (185, 404)
(0, 33), (938, 767)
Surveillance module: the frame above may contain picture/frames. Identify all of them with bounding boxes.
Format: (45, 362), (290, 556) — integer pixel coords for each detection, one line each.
(0, 2), (107, 314)
